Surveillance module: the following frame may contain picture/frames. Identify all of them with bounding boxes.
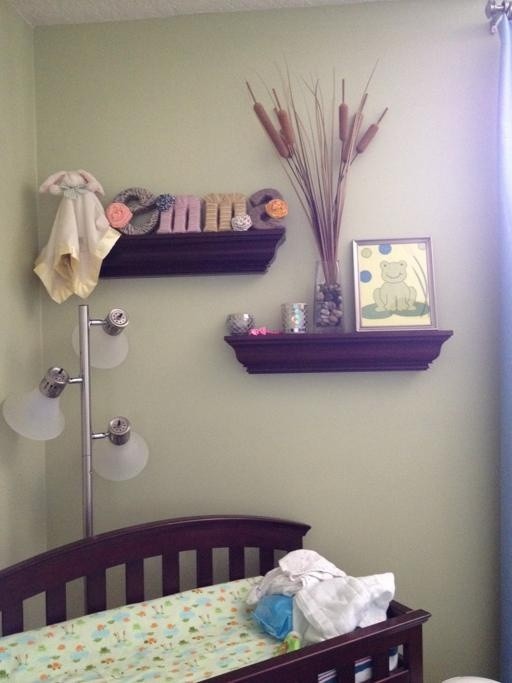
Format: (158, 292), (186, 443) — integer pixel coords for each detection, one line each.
(351, 236), (440, 333)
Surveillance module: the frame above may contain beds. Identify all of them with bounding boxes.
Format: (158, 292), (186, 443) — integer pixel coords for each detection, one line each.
(3, 515), (431, 683)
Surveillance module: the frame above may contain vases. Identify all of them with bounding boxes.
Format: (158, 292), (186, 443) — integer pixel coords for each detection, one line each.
(313, 258), (347, 334)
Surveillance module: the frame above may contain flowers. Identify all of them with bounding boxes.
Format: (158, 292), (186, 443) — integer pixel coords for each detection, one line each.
(242, 57), (389, 286)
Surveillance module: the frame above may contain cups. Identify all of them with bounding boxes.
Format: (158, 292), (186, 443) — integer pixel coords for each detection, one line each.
(281, 302), (306, 331)
(225, 312), (254, 334)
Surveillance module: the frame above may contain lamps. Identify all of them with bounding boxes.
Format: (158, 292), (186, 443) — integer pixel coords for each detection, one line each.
(2, 304), (148, 541)
